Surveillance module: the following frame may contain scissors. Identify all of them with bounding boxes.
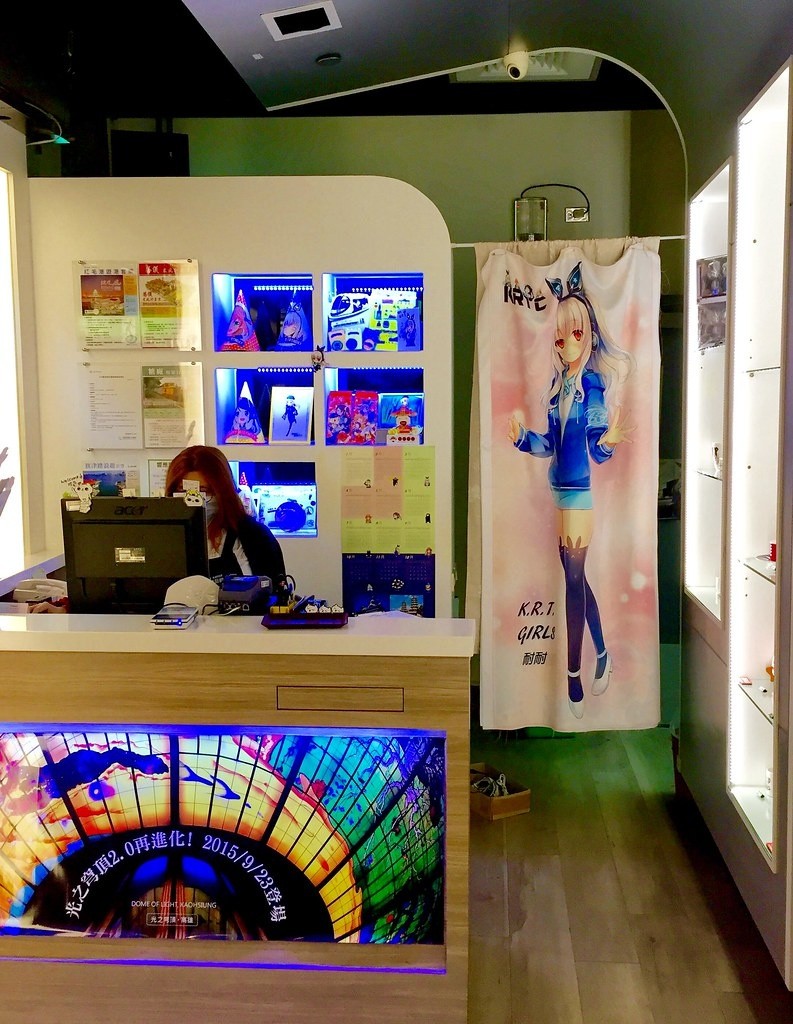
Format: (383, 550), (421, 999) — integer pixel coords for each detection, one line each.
(277, 573), (296, 600)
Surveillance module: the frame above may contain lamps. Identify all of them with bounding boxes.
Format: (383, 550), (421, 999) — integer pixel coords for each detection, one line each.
(514, 182), (592, 242)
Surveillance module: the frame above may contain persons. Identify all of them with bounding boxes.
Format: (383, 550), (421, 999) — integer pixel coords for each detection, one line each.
(28, 445), (289, 616)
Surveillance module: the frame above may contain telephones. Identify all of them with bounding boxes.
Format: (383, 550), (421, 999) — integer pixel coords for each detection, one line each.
(12, 578), (68, 604)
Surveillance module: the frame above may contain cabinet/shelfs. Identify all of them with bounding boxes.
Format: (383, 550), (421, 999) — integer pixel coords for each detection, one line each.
(672, 53), (792, 982)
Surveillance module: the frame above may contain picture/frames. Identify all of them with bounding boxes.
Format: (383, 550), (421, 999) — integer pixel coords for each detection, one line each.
(268, 385), (315, 446)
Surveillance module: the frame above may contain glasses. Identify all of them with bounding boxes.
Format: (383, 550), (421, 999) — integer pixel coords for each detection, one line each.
(199, 486), (221, 503)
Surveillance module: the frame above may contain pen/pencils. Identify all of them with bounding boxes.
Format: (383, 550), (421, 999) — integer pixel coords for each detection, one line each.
(274, 582), (293, 606)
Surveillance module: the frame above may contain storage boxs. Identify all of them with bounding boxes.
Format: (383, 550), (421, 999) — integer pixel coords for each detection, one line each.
(471, 762), (531, 820)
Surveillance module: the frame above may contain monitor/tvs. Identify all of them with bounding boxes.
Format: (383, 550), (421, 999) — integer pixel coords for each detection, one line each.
(59, 497), (210, 615)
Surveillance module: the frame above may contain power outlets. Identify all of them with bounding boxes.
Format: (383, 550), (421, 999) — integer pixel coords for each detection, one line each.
(565, 208), (589, 222)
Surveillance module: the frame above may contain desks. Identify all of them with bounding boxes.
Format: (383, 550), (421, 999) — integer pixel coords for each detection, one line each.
(0, 605), (477, 1024)
